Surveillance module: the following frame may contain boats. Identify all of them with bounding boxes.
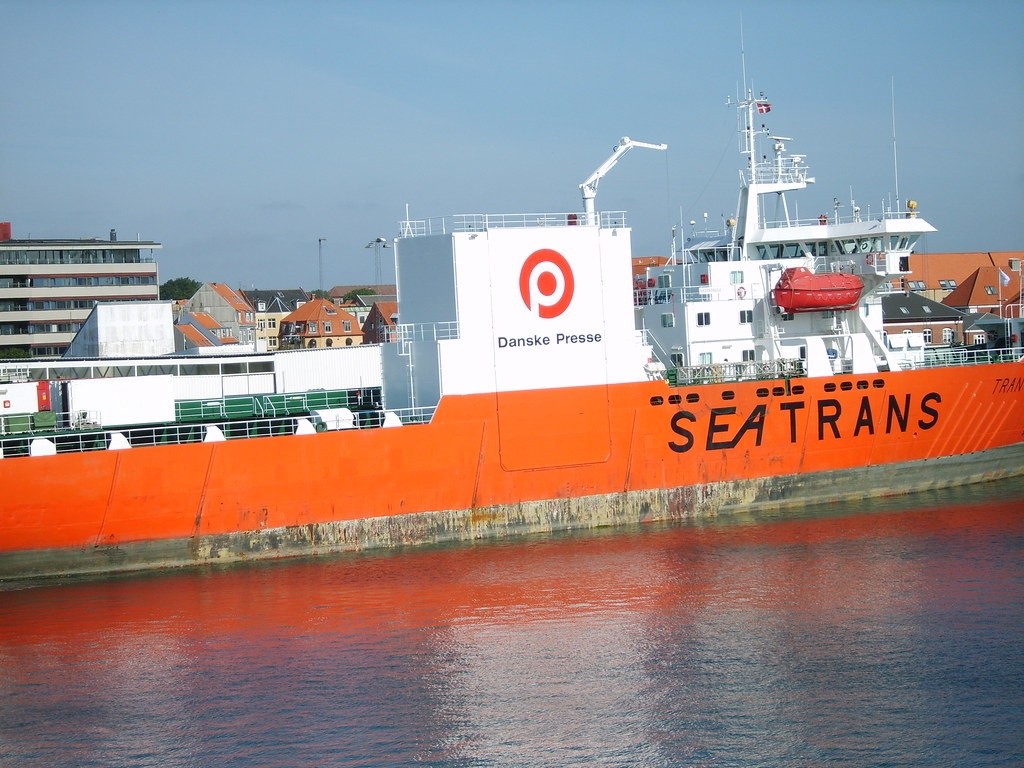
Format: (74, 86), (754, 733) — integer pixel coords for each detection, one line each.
(772, 267), (865, 313)
(0, 85), (1024, 591)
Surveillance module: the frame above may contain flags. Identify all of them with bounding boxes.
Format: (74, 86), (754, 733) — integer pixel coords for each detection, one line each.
(756, 103), (772, 113)
